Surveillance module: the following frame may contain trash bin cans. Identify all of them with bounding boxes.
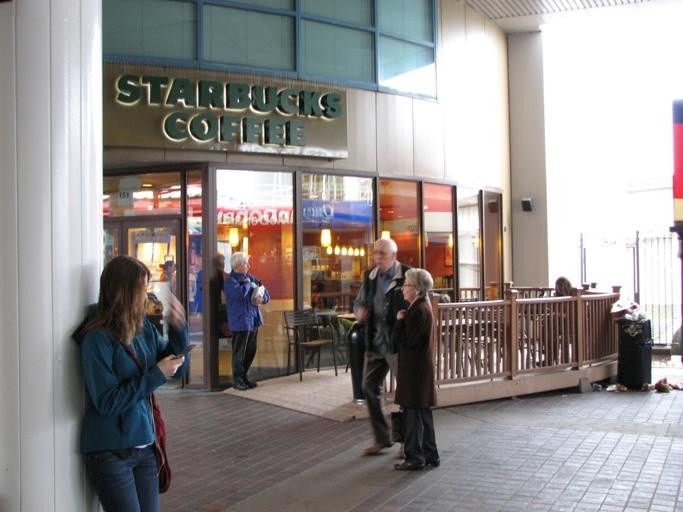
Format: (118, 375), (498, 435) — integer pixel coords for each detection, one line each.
(618, 319), (651, 390)
(347, 322), (385, 405)
(146, 292), (163, 336)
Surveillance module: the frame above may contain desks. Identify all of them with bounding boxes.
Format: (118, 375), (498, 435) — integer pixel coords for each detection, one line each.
(437, 307), (561, 377)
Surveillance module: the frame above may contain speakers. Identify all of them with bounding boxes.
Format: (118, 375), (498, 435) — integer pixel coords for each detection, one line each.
(488, 199), (497, 213)
(521, 198), (533, 213)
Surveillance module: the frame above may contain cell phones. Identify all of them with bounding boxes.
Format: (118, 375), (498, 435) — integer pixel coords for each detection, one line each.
(170, 344), (196, 361)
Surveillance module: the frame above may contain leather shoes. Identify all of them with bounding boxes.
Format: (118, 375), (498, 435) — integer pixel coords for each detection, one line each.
(394, 443), (440, 471)
(232, 381), (256, 390)
(362, 438), (395, 455)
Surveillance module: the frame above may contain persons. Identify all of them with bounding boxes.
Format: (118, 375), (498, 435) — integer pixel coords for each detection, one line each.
(386, 263), (441, 470)
(69, 259), (194, 512)
(535, 276), (576, 368)
(225, 251), (269, 389)
(159, 260), (177, 294)
(193, 256), (226, 314)
(354, 239), (415, 456)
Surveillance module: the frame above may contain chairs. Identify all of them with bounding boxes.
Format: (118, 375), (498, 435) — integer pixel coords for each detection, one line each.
(339, 317), (355, 372)
(283, 308), (340, 382)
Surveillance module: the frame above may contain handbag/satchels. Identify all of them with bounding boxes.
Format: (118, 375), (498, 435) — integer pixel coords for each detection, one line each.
(152, 404), (171, 494)
(390, 411), (407, 443)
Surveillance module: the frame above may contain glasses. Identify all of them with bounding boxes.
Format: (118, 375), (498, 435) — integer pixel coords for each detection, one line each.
(400, 284), (419, 288)
